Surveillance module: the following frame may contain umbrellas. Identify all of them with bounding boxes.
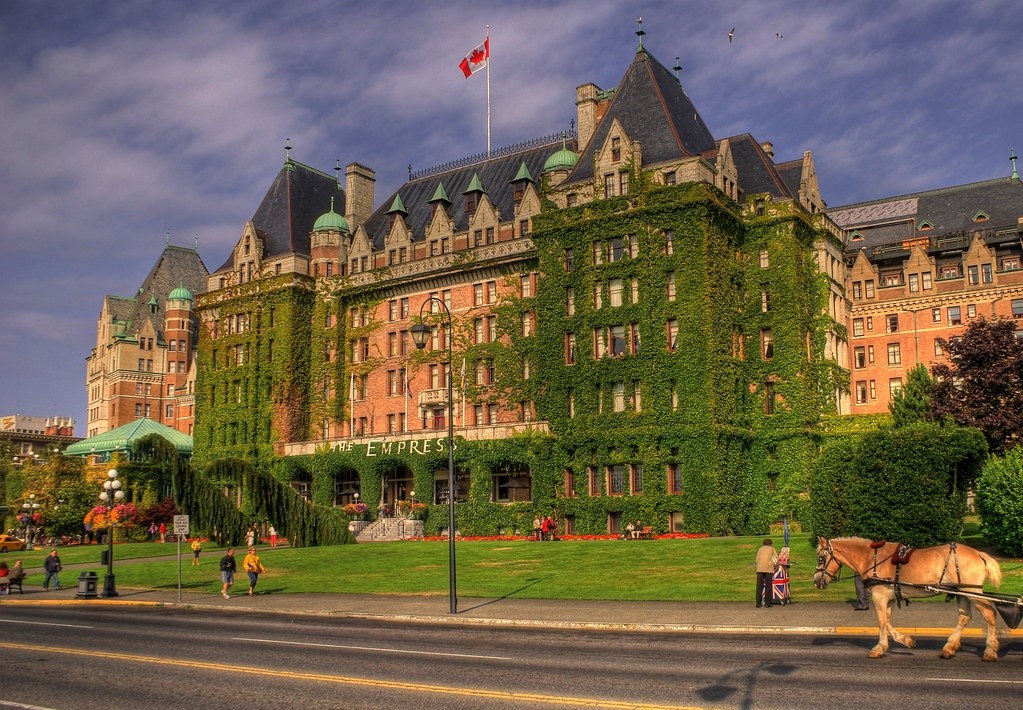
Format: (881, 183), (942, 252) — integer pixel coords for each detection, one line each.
(338, 488), (361, 504)
(500, 479), (530, 501)
(783, 518), (790, 547)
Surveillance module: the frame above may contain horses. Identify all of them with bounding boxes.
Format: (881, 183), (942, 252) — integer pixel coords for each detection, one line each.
(812, 535), (1002, 661)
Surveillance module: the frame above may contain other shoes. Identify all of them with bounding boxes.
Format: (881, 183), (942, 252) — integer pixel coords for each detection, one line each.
(221, 590), (224, 593)
(45, 587), (49, 591)
(756, 604), (761, 607)
(55, 587), (62, 592)
(196, 563), (199, 565)
(765, 604), (772, 608)
(249, 593), (256, 597)
(223, 594), (231, 599)
(192, 563), (195, 565)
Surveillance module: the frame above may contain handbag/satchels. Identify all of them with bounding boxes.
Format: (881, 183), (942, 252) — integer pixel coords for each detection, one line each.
(257, 566), (262, 573)
(56, 565), (63, 572)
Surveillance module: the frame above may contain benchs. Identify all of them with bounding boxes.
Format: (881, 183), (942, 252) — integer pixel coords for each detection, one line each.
(527, 530), (535, 541)
(0, 573), (27, 595)
(641, 527), (653, 540)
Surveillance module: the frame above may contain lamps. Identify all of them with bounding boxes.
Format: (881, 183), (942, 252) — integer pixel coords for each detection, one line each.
(409, 490), (416, 503)
(354, 492), (359, 504)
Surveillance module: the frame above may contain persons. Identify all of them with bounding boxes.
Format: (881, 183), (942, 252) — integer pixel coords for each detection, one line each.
(159, 523), (167, 543)
(190, 537), (201, 566)
(87, 531), (94, 544)
(9, 560), (24, 577)
(853, 572), (870, 611)
(630, 521), (643, 540)
(532, 515), (555, 541)
(244, 527), (254, 547)
(269, 524), (277, 547)
(181, 534), (187, 543)
(43, 550), (64, 591)
(149, 522), (158, 541)
(621, 522), (634, 540)
(0, 562), (10, 577)
(755, 538), (782, 609)
(243, 547), (266, 596)
(219, 548), (237, 599)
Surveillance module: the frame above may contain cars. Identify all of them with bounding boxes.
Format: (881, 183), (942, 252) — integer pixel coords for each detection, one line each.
(0, 534), (27, 554)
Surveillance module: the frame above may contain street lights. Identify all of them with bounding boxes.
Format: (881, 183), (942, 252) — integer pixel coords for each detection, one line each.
(409, 491), (415, 510)
(406, 298), (458, 616)
(353, 492), (359, 505)
(100, 467), (125, 598)
(21, 493), (40, 549)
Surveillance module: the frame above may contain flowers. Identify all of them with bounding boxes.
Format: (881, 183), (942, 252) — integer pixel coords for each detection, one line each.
(411, 503), (428, 520)
(399, 500), (412, 514)
(341, 503), (358, 514)
(353, 503), (367, 514)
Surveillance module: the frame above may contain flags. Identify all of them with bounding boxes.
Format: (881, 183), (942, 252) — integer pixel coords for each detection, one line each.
(459, 35), (490, 80)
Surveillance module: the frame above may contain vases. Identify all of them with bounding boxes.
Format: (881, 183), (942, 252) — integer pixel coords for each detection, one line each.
(411, 513), (420, 520)
(357, 513), (365, 521)
(351, 514), (358, 521)
(403, 510), (409, 518)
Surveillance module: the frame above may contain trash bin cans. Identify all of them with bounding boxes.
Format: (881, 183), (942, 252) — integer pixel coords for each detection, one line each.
(76, 572), (99, 599)
